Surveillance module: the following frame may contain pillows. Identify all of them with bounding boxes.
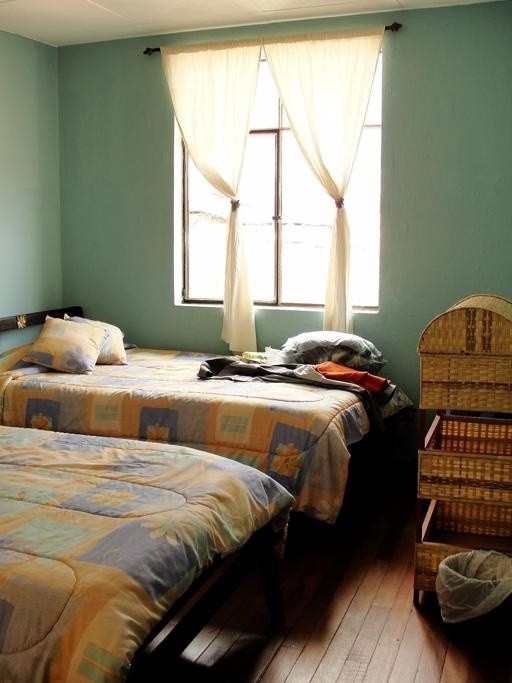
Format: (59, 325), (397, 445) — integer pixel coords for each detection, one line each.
(24, 314), (110, 373)
(66, 311), (128, 368)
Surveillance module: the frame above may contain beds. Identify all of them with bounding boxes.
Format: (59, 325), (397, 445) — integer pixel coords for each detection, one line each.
(1, 423), (293, 683)
(1, 304), (414, 538)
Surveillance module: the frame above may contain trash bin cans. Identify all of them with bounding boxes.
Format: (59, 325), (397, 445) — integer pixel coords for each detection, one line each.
(434, 550), (512, 654)
